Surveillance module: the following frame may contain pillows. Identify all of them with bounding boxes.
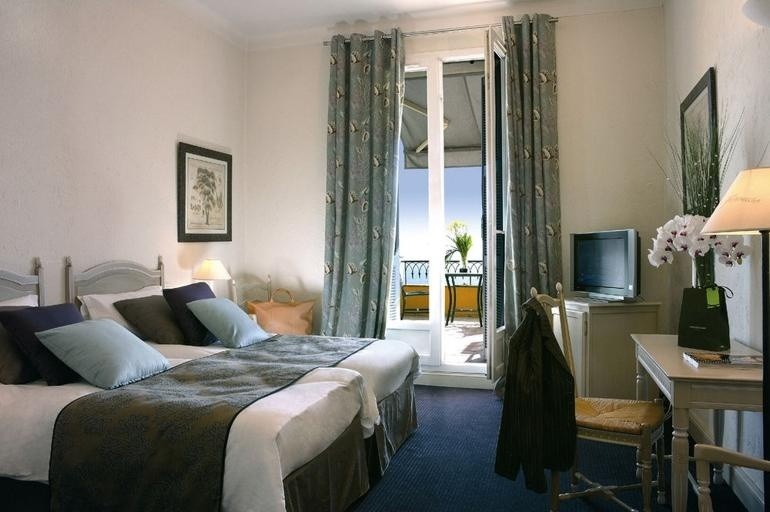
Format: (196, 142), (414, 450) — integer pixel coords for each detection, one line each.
(2, 282), (278, 391)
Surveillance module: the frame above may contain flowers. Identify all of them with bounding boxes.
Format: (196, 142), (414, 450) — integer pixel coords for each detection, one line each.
(445, 217), (474, 268)
(647, 97), (756, 286)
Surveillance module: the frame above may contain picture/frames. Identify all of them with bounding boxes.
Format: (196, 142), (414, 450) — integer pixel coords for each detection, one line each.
(176, 140), (233, 244)
(678, 66), (722, 219)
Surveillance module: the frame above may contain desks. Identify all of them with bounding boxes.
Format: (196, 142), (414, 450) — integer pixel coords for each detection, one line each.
(630, 333), (764, 512)
(445, 272), (484, 328)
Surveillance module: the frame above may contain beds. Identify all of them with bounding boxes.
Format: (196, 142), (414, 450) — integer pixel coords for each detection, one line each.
(2, 253), (382, 511)
(64, 254), (423, 481)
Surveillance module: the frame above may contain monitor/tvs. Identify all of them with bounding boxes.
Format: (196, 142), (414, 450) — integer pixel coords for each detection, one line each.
(569, 228), (641, 302)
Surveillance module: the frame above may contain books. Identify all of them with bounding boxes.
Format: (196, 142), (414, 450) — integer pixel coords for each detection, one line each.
(683, 352), (763, 369)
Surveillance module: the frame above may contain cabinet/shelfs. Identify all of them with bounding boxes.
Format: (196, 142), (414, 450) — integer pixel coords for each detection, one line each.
(546, 298), (662, 399)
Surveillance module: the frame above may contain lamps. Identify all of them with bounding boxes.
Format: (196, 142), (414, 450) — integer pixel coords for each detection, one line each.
(192, 258), (233, 292)
(699, 165), (770, 512)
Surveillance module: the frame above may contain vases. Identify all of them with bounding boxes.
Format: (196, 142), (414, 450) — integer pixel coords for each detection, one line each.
(458, 255), (469, 272)
(690, 249), (716, 292)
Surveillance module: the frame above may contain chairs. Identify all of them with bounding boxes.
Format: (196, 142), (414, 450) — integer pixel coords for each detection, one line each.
(230, 273), (273, 316)
(400, 281), (430, 320)
(522, 281), (669, 512)
